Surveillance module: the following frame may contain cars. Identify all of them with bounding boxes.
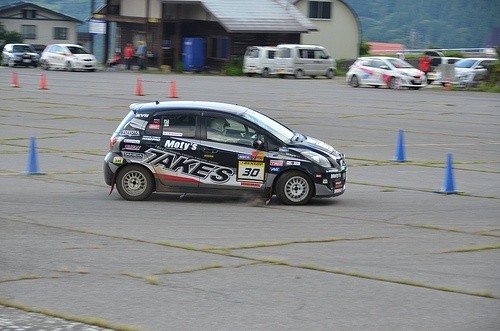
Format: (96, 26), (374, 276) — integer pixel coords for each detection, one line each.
(103, 100), (349, 206)
(39, 43), (98, 72)
(346, 56), (427, 90)
(0, 43), (46, 68)
(401, 55), (499, 87)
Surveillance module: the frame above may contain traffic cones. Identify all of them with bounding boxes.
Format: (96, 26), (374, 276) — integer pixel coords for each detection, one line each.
(167, 78), (180, 98)
(37, 70), (50, 90)
(9, 70), (21, 87)
(20, 135), (47, 175)
(132, 78), (145, 96)
(388, 128), (412, 162)
(434, 152), (465, 194)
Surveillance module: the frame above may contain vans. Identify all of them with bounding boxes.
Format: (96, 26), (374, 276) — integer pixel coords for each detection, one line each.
(278, 44), (337, 80)
(242, 45), (279, 78)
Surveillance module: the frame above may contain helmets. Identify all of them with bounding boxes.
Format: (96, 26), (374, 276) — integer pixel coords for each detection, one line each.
(210, 118), (230, 135)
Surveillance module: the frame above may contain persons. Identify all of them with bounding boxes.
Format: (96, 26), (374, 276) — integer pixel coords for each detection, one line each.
(207, 119), (233, 142)
(105, 39), (147, 71)
(418, 52), (431, 77)
